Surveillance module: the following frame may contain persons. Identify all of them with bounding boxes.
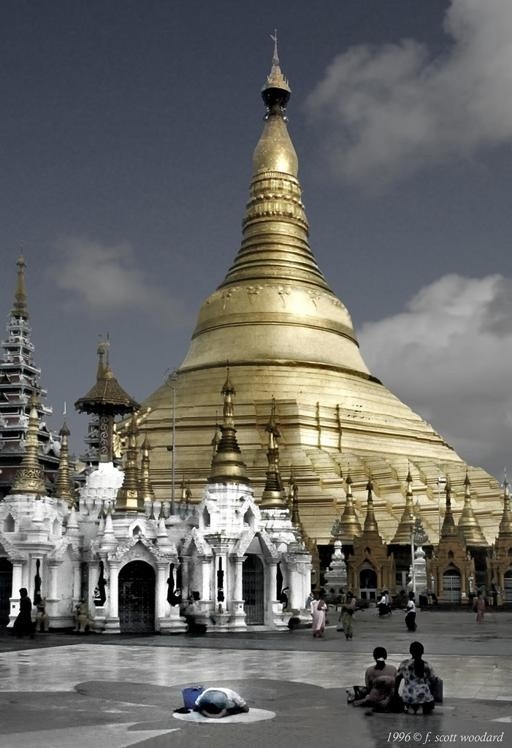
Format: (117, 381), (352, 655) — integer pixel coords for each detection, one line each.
(339, 590), (359, 640)
(366, 647), (397, 699)
(309, 591), (328, 637)
(13, 588), (35, 639)
(474, 594), (487, 623)
(396, 641), (438, 717)
(375, 590), (395, 617)
(196, 687), (249, 719)
(336, 593), (346, 632)
(404, 590), (418, 631)
